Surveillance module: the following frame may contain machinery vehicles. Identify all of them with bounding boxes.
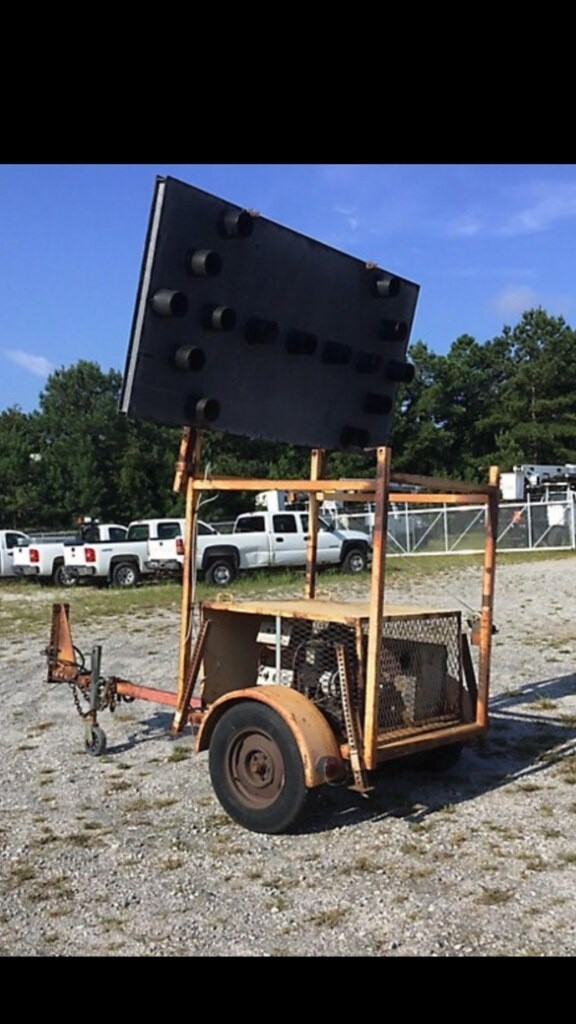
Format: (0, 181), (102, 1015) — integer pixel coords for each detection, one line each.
(256, 483), (430, 550)
(494, 464), (576, 547)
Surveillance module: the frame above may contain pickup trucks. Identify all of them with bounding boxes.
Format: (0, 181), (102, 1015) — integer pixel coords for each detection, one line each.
(12, 523), (127, 589)
(63, 519), (222, 589)
(0, 529), (31, 577)
(176, 510), (372, 586)
